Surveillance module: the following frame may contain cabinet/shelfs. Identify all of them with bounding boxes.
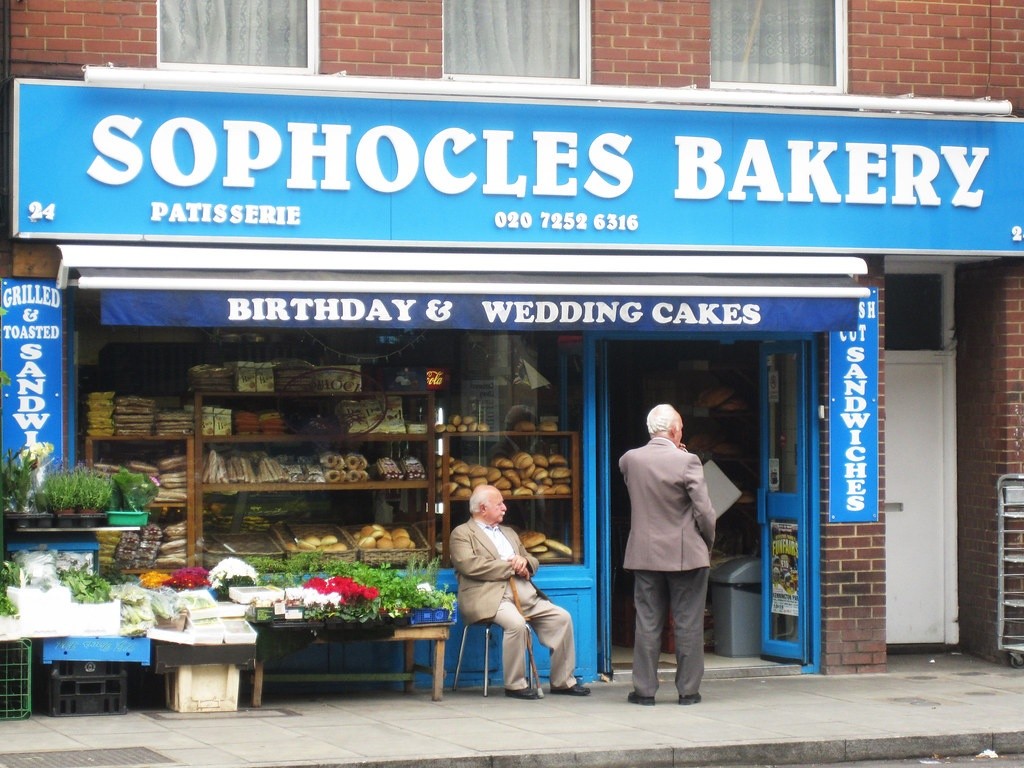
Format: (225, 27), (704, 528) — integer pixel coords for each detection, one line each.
(997, 473), (1024, 668)
(193, 390), (436, 569)
(437, 432), (580, 565)
(682, 362), (760, 517)
(87, 434), (194, 573)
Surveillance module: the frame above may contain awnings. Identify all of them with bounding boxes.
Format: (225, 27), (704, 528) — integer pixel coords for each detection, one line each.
(82, 277), (870, 332)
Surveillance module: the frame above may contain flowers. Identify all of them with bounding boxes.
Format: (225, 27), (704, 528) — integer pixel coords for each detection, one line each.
(305, 575), (380, 623)
(109, 463), (161, 513)
(0, 441), (54, 513)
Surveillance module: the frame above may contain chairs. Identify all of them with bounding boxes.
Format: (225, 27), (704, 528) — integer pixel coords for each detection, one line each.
(452, 574), (534, 697)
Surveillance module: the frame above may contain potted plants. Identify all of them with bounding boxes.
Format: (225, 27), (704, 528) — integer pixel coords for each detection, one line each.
(44, 457), (113, 528)
(379, 553), (458, 625)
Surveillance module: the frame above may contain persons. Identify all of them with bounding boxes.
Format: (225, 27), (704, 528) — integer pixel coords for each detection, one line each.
(448, 485), (591, 700)
(619, 403), (717, 706)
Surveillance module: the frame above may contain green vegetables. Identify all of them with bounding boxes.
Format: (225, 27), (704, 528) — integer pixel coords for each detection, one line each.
(0, 559), (110, 615)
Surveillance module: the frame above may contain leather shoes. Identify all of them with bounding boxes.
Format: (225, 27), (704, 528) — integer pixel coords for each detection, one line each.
(550, 684), (591, 696)
(627, 692), (655, 707)
(679, 693), (703, 705)
(504, 687), (544, 700)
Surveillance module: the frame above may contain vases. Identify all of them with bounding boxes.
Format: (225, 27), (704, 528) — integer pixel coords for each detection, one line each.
(107, 510), (148, 526)
(326, 612), (380, 625)
(5, 513), (51, 527)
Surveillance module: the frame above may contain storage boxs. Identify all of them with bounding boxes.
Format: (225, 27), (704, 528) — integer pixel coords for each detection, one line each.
(0, 586), (285, 720)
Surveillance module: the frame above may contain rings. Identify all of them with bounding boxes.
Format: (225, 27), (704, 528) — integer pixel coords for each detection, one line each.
(520, 562), (523, 565)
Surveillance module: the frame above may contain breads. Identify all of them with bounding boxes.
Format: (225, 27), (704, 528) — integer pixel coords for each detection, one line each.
(287, 524), (415, 551)
(504, 524), (572, 559)
(514, 421), (558, 431)
(94, 449), (287, 502)
(436, 414), (489, 432)
(436, 450), (572, 497)
(155, 519), (187, 570)
(688, 385), (750, 455)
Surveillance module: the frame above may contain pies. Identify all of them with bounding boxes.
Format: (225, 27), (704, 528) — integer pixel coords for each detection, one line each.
(113, 397), (195, 436)
(188, 369), (315, 392)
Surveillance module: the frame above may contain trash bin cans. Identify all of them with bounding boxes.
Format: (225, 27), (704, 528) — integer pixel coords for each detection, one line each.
(708, 556), (762, 658)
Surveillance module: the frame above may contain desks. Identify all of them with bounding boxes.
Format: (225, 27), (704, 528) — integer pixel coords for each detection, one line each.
(250, 625), (449, 701)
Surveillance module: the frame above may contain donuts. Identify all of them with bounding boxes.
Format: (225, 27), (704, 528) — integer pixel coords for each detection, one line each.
(322, 452), (369, 482)
